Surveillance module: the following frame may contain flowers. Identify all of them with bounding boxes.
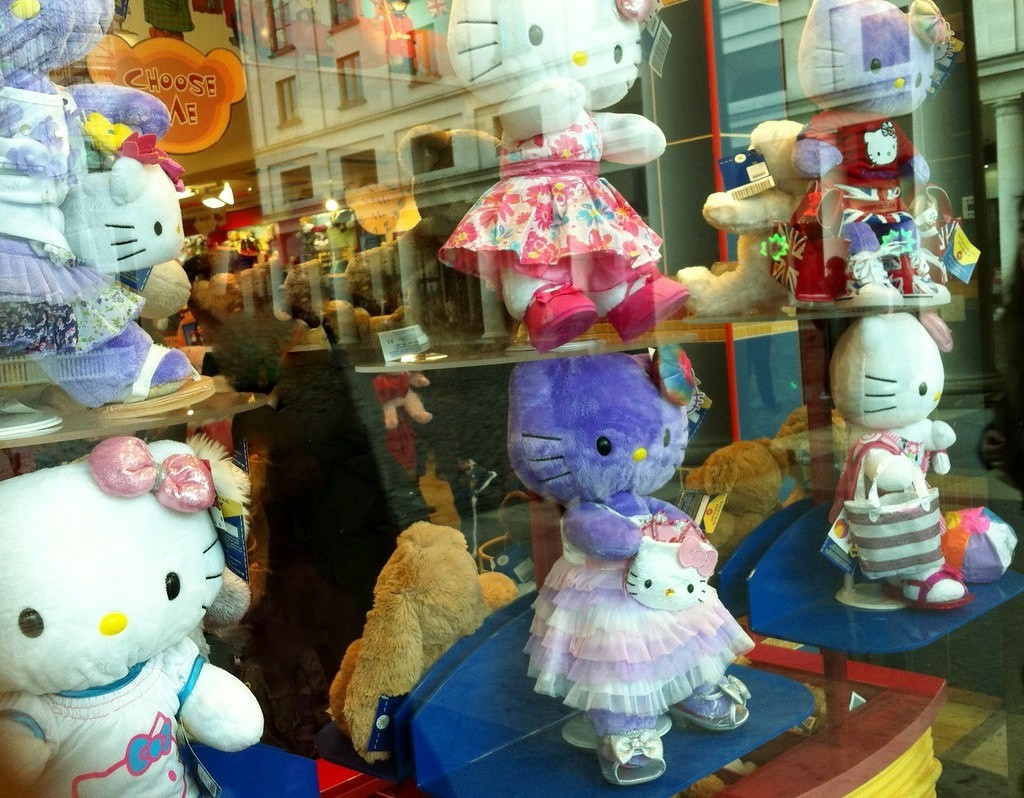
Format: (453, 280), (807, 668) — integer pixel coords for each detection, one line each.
(85, 112), (186, 192)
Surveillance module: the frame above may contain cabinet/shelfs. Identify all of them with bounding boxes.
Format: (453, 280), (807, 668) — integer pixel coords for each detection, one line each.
(0, 304), (1024, 797)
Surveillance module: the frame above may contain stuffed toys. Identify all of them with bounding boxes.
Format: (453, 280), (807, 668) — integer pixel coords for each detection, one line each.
(0, 0), (1024, 798)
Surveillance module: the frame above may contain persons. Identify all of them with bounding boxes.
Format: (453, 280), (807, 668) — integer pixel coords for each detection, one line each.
(183, 240), (213, 284)
(976, 192), (1024, 491)
(751, 336), (780, 407)
